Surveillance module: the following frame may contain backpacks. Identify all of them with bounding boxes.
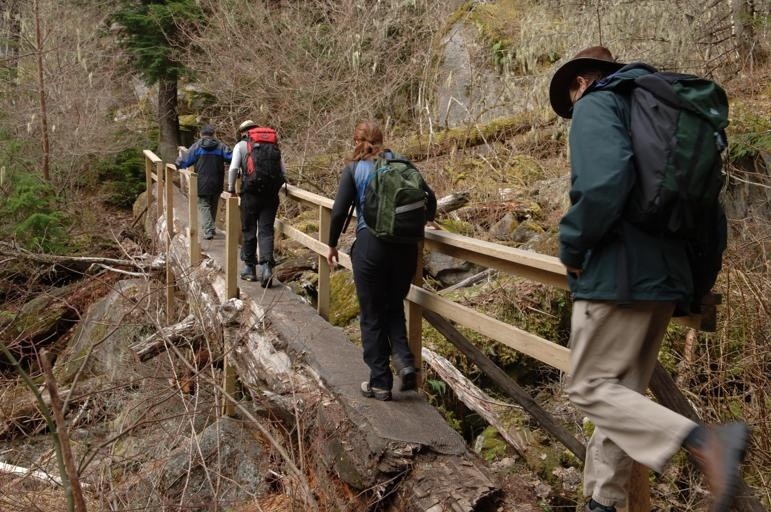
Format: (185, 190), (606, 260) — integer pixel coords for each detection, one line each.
(343, 155), (438, 245)
(613, 71), (732, 307)
(244, 127), (288, 198)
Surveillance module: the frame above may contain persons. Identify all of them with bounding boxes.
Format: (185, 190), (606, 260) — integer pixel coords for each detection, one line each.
(223, 122), (285, 287)
(542, 43), (750, 510)
(326, 122), (417, 397)
(178, 129), (231, 241)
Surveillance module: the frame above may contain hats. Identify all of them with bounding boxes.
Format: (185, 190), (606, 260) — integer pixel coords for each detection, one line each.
(549, 44), (631, 118)
(238, 120), (259, 132)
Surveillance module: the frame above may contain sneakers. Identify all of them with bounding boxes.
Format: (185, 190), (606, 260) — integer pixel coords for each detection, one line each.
(209, 229), (215, 235)
(202, 231), (213, 240)
(398, 367), (419, 393)
(360, 380), (394, 402)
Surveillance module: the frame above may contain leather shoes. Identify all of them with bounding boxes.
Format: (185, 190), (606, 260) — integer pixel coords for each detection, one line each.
(691, 421), (750, 511)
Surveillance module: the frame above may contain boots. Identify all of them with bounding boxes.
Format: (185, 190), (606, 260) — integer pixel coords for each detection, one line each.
(259, 262), (273, 288)
(240, 263), (257, 282)
